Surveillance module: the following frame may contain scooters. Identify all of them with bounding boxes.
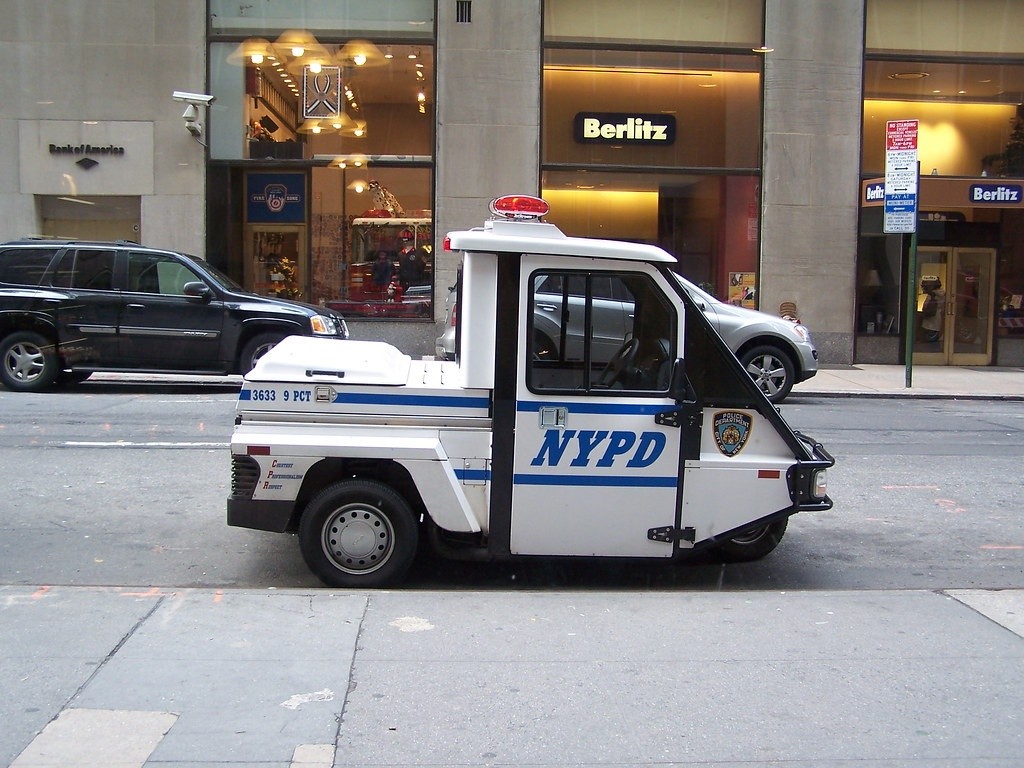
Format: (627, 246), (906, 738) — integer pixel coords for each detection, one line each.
(220, 192), (837, 589)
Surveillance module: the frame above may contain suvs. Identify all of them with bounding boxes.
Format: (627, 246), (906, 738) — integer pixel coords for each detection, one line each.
(0, 234), (350, 394)
(433, 269), (820, 404)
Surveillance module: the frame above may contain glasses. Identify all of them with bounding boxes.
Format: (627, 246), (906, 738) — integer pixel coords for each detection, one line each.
(401, 238), (414, 242)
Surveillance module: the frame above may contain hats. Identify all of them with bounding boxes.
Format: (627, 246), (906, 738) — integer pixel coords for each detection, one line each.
(399, 229), (413, 239)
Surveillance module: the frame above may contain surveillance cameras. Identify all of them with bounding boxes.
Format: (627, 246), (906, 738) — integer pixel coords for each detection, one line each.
(172, 91), (216, 107)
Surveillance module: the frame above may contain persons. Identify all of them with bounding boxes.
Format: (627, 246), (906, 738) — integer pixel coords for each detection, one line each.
(372, 229), (428, 305)
(918, 278), (946, 343)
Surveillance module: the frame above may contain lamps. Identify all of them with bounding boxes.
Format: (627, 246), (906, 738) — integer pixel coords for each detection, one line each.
(226, 29), (389, 75)
(296, 111), (367, 138)
(328, 151), (368, 170)
(347, 179), (371, 193)
